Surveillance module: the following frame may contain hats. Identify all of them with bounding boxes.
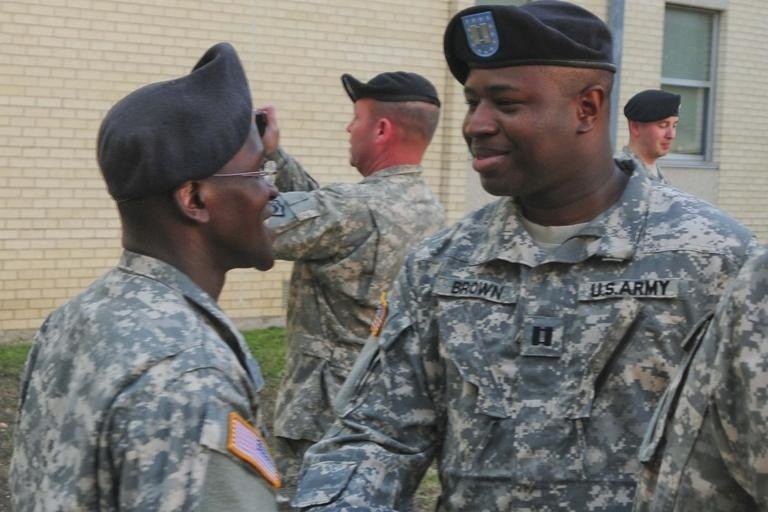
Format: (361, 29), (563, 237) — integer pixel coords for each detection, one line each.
(624, 90), (681, 121)
(97, 43), (251, 204)
(445, 1), (617, 85)
(342, 72), (440, 107)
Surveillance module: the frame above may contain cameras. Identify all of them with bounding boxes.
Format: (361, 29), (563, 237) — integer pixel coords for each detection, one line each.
(255, 113), (268, 136)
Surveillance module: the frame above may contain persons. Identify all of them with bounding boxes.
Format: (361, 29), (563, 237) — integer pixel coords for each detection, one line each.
(612, 90), (681, 187)
(285, 0), (767, 511)
(8, 42), (282, 512)
(253, 71), (448, 512)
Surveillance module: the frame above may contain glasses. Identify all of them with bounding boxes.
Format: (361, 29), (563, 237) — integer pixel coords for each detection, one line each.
(212, 160), (278, 187)
